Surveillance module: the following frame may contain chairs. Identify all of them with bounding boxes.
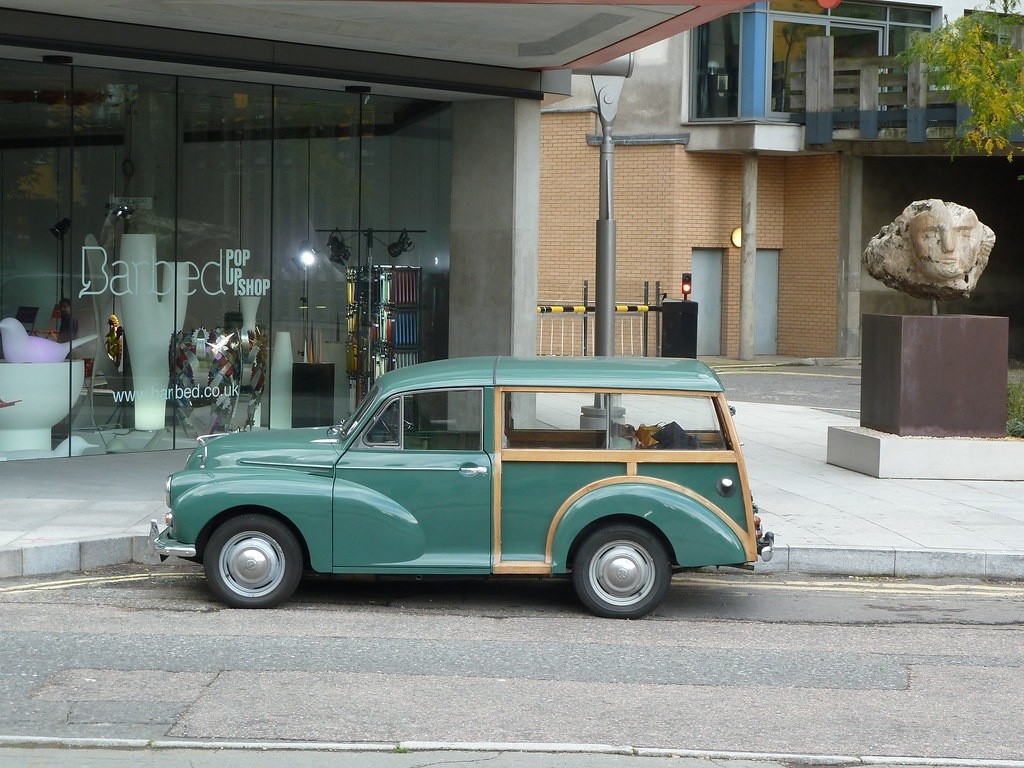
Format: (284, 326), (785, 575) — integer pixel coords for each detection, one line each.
(31, 304), (61, 340)
(14, 306), (40, 335)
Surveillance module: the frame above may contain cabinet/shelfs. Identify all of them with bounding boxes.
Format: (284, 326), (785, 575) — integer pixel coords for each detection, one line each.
(347, 265), (451, 442)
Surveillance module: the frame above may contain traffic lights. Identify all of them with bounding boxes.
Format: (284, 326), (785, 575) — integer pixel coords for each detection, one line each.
(681, 273), (692, 294)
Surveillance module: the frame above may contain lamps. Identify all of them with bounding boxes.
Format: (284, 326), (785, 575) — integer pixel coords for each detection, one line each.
(298, 240), (322, 266)
(326, 228), (351, 265)
(387, 227), (415, 257)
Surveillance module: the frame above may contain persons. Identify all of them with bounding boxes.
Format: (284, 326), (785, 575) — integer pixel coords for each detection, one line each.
(909, 199), (981, 279)
(57, 298), (78, 344)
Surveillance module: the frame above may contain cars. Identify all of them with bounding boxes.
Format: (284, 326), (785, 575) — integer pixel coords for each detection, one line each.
(145, 351), (778, 621)
